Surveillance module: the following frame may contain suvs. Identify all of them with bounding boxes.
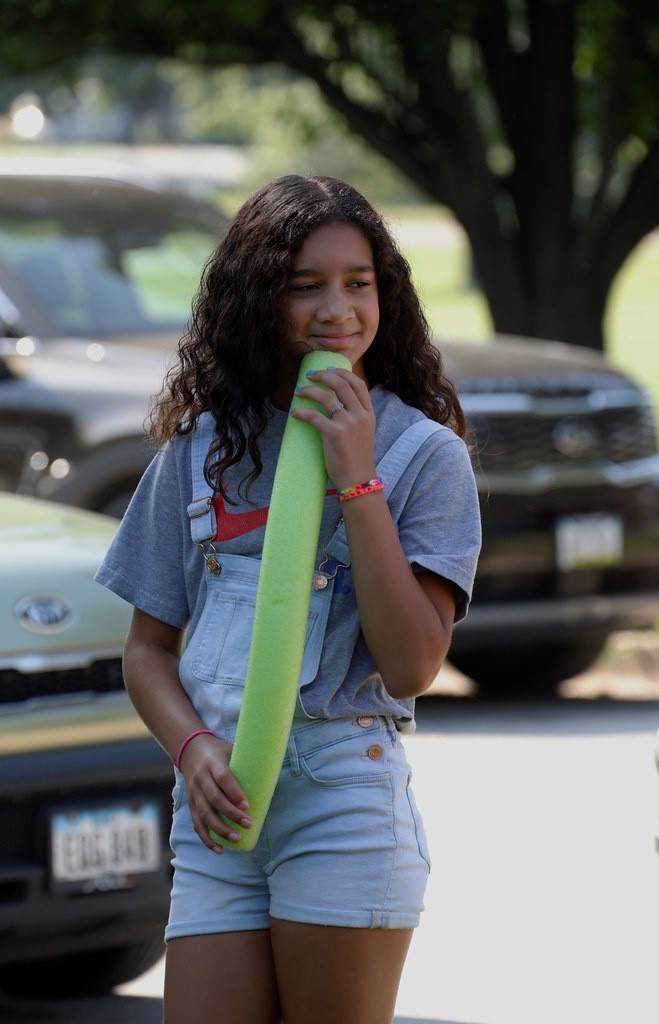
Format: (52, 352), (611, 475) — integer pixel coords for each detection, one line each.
(1, 170), (658, 693)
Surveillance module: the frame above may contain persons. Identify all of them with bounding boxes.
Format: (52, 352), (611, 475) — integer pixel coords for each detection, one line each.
(96, 176), (483, 1023)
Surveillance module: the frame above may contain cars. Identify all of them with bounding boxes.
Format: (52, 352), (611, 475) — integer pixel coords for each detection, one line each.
(2, 489), (177, 996)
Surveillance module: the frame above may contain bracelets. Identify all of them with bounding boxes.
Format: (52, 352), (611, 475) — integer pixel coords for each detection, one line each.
(176, 727), (223, 775)
(336, 473), (387, 502)
